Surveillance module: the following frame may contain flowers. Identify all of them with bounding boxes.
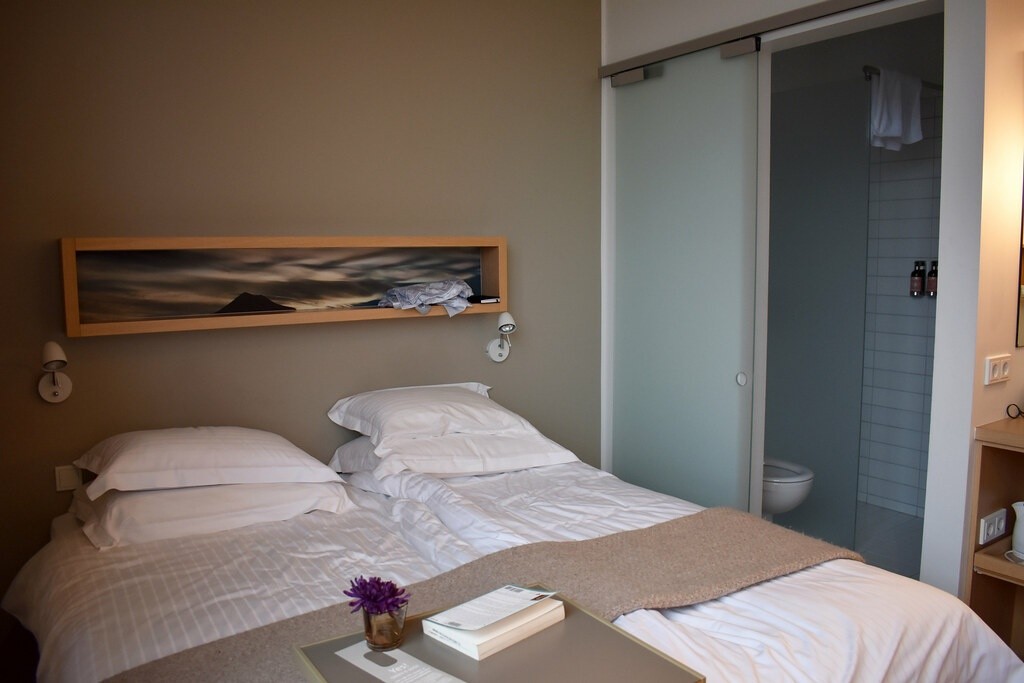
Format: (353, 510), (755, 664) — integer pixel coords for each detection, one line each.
(341, 574), (410, 616)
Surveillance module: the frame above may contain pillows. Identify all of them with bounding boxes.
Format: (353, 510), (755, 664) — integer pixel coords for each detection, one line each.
(328, 429), (582, 481)
(326, 381), (540, 460)
(67, 481), (362, 550)
(72, 425), (348, 501)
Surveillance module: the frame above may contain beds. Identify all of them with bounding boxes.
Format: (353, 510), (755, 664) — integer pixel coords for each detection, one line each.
(2, 463), (1024, 683)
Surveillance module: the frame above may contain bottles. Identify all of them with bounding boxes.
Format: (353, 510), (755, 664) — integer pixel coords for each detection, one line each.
(927, 260), (937, 299)
(909, 261), (925, 297)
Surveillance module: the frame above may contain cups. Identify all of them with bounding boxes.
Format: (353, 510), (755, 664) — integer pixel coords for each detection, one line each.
(363, 601), (408, 652)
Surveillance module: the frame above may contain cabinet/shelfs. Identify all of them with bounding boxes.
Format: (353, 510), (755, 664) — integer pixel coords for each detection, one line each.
(961, 439), (1024, 662)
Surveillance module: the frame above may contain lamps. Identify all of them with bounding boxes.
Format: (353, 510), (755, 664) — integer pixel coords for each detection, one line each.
(485, 311), (516, 363)
(38, 340), (73, 404)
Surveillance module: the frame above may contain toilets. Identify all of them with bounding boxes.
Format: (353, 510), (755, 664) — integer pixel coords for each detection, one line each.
(762, 456), (814, 522)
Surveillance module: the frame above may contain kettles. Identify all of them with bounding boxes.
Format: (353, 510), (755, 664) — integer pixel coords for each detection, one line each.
(1011, 501), (1024, 560)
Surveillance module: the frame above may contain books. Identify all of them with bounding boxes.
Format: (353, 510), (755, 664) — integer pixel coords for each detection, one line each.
(422, 585), (565, 661)
(467, 295), (501, 304)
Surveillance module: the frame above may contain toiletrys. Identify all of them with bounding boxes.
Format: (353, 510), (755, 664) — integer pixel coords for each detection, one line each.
(926, 261), (938, 297)
(908, 260), (925, 298)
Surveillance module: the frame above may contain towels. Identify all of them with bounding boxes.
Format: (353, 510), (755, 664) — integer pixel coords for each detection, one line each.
(378, 279), (474, 318)
(870, 63), (923, 150)
